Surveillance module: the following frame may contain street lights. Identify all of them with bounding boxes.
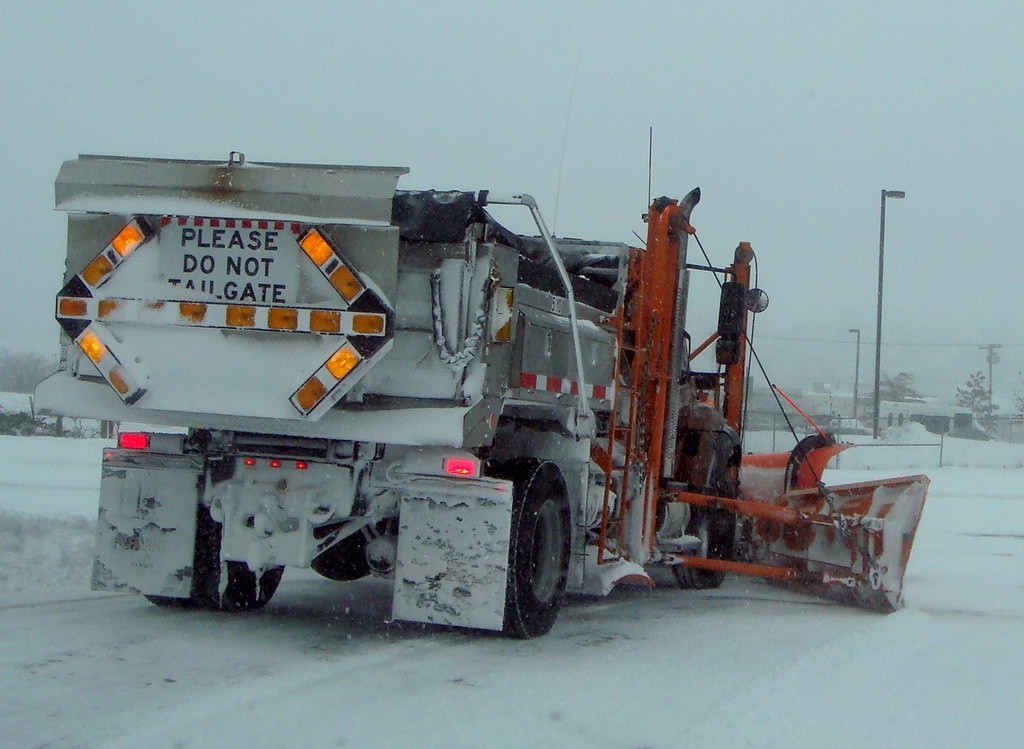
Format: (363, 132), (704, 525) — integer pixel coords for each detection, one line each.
(848, 329), (861, 418)
(869, 188), (907, 439)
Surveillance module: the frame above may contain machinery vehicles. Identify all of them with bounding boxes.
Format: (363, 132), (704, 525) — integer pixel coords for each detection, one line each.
(28, 149), (931, 640)
